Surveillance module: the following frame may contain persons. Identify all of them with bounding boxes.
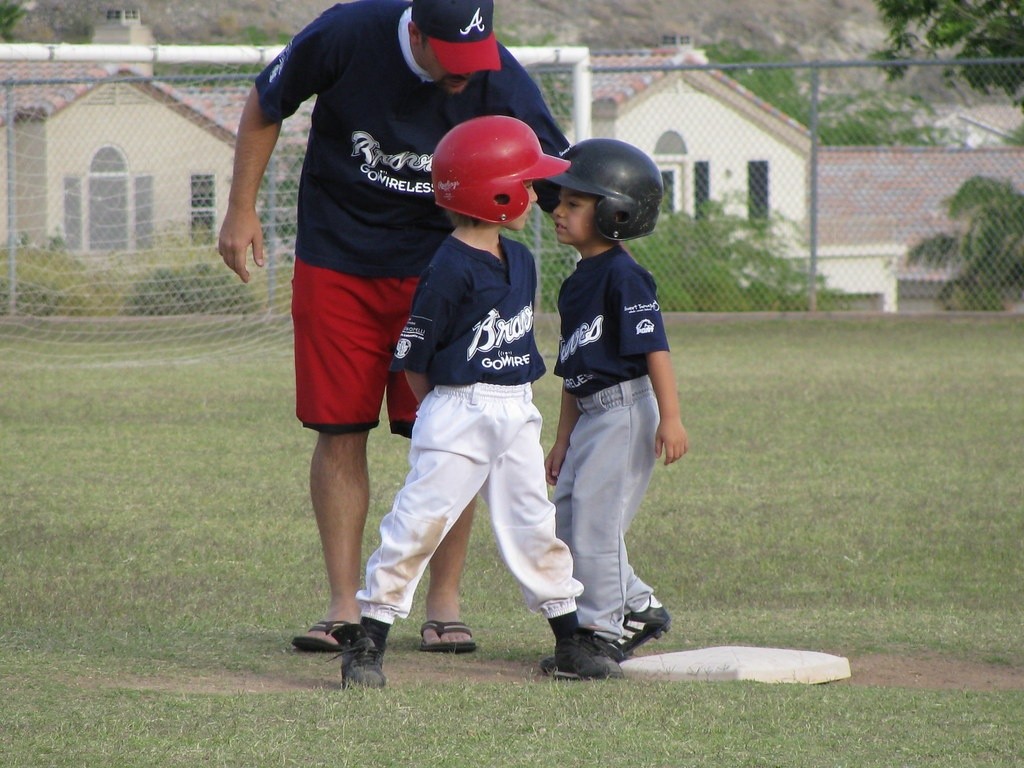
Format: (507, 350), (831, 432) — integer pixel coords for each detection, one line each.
(340, 115), (623, 689)
(220, 0), (571, 653)
(540, 137), (688, 676)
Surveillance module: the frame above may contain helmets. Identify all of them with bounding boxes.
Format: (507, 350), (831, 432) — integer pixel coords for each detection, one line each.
(544, 138), (663, 241)
(431, 116), (572, 223)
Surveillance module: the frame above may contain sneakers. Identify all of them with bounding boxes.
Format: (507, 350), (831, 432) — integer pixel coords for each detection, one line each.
(331, 623), (390, 689)
(553, 625), (624, 680)
(541, 595), (671, 674)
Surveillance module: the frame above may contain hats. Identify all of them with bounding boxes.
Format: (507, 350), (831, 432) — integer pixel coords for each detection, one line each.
(412, 0), (502, 74)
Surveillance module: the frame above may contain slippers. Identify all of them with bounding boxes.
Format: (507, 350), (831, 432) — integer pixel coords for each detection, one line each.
(292, 620), (351, 651)
(420, 620), (476, 651)
